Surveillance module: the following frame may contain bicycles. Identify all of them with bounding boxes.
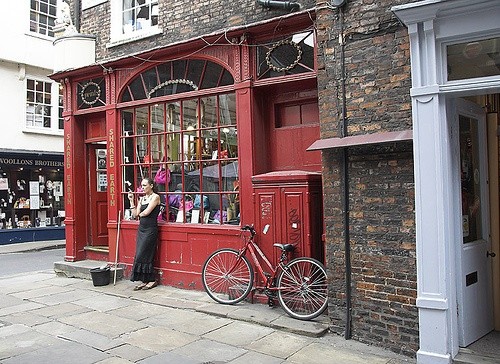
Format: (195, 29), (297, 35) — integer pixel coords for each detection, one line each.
(203, 224), (331, 320)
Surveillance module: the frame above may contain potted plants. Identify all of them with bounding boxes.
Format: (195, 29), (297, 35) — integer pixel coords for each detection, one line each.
(224, 199), (233, 222)
(228, 187), (237, 219)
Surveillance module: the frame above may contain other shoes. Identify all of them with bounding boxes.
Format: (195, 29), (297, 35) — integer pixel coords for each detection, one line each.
(143, 281), (157, 289)
(133, 285), (145, 290)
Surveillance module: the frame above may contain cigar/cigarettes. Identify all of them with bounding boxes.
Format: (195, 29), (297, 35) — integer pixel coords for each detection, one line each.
(128, 188), (132, 192)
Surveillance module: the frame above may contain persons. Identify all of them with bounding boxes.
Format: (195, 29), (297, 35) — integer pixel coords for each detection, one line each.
(128, 178), (161, 291)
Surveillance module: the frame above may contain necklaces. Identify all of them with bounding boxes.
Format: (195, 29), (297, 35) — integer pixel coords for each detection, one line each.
(144, 192), (153, 201)
